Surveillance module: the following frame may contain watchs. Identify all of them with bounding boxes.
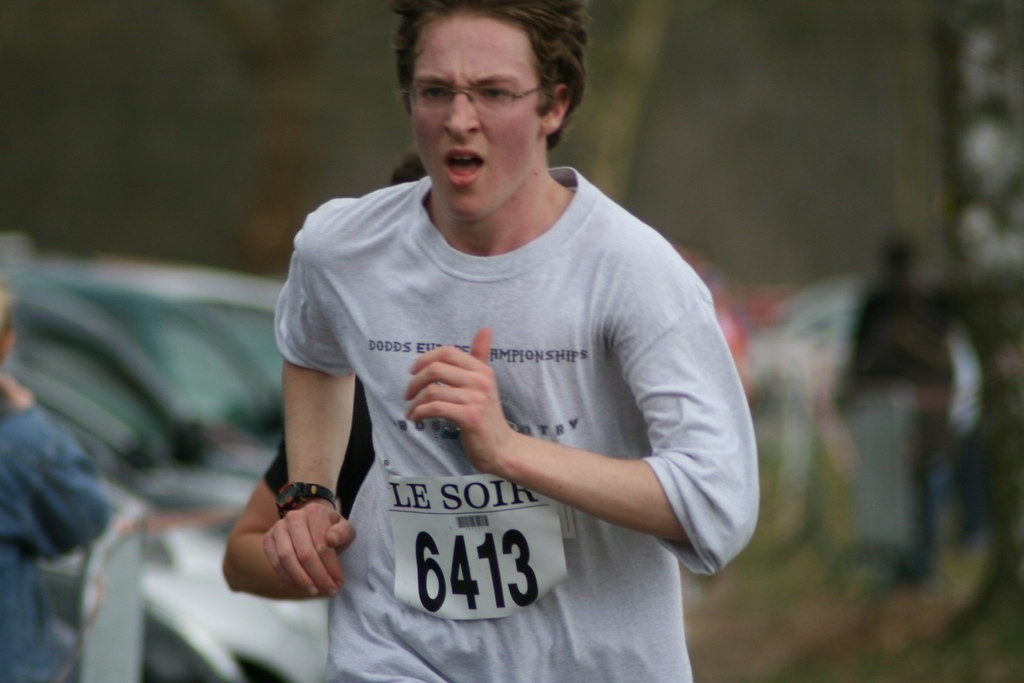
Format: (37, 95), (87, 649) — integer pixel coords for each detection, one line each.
(275, 482), (336, 517)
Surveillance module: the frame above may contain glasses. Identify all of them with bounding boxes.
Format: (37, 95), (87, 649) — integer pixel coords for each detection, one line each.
(410, 82), (542, 118)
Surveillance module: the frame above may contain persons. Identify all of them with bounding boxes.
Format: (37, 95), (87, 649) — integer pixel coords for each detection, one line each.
(222, 0), (759, 683)
(830, 229), (992, 584)
(0, 279), (111, 683)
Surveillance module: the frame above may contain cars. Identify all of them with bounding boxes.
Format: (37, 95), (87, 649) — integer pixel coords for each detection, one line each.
(0, 232), (791, 683)
